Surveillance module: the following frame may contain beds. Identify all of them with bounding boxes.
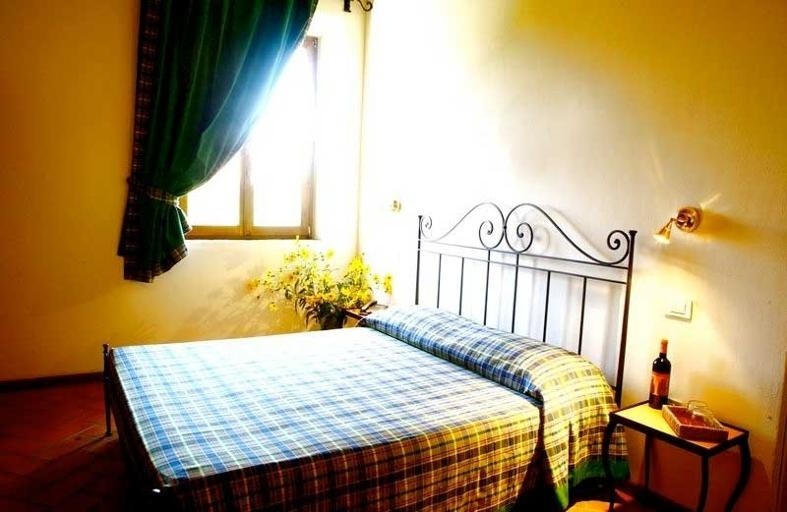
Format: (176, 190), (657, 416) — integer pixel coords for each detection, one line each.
(102, 200), (637, 512)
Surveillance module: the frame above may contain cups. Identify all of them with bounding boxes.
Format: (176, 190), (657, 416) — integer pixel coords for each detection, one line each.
(686, 400), (713, 427)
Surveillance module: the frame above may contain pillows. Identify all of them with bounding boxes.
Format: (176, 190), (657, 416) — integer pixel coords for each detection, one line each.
(364, 304), (614, 401)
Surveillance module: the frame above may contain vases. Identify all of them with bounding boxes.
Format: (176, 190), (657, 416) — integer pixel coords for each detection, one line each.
(318, 313), (345, 330)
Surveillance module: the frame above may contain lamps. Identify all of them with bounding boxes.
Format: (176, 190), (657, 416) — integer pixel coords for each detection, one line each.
(651, 204), (701, 246)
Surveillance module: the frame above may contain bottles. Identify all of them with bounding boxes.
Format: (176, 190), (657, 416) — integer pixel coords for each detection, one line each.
(649, 338), (671, 410)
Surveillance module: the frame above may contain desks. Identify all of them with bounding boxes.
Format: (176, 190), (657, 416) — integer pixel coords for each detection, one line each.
(601, 397), (749, 512)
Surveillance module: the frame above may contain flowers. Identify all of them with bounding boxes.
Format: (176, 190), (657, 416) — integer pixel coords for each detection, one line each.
(246, 235), (393, 329)
